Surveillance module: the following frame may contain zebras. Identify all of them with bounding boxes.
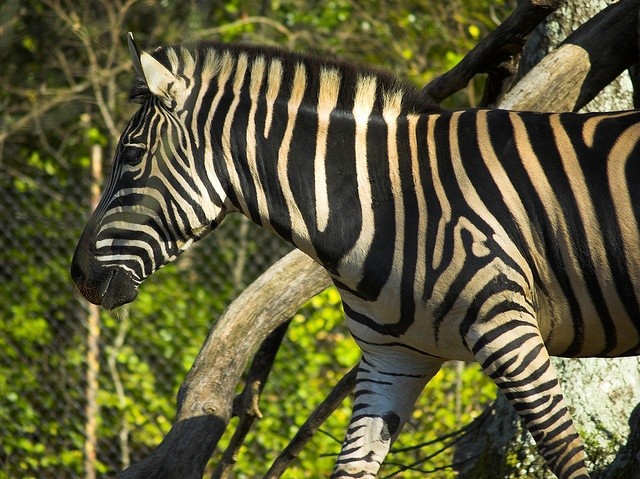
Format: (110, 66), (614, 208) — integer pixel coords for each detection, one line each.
(70, 30), (640, 479)
